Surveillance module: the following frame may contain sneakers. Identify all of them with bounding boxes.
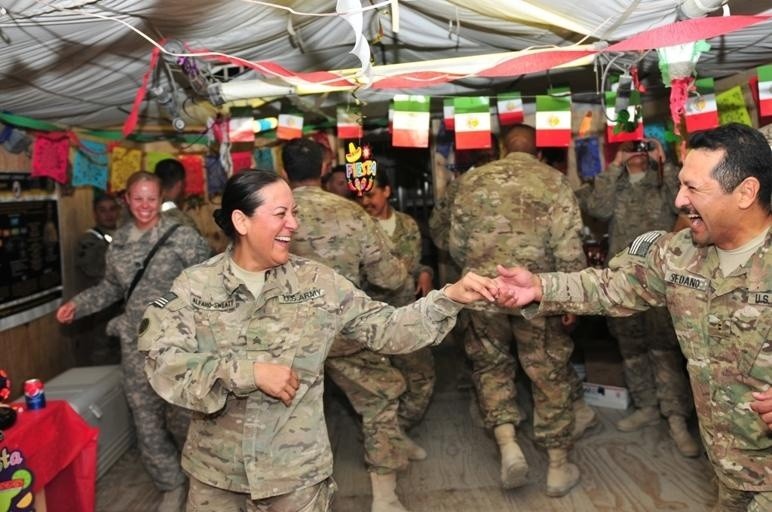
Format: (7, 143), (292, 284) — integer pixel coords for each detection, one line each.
(615, 404), (662, 433)
(159, 483), (187, 512)
(401, 429), (427, 460)
(667, 414), (700, 457)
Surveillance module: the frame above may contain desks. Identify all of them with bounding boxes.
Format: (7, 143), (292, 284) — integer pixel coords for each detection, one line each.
(0, 395), (100, 512)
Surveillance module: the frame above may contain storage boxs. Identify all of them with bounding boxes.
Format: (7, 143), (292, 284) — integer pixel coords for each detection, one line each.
(11, 363), (137, 485)
(581, 382), (633, 411)
(584, 346), (627, 389)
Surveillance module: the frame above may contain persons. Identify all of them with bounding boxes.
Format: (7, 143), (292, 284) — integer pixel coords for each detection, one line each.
(56, 138), (498, 511)
(451, 126), (772, 510)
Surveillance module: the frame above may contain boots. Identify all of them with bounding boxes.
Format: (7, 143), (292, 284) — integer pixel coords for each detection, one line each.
(493, 422), (530, 490)
(369, 469), (411, 512)
(545, 446), (580, 498)
(571, 395), (600, 440)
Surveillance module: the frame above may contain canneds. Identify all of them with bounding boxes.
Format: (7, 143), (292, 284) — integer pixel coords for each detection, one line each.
(23, 378), (46, 410)
